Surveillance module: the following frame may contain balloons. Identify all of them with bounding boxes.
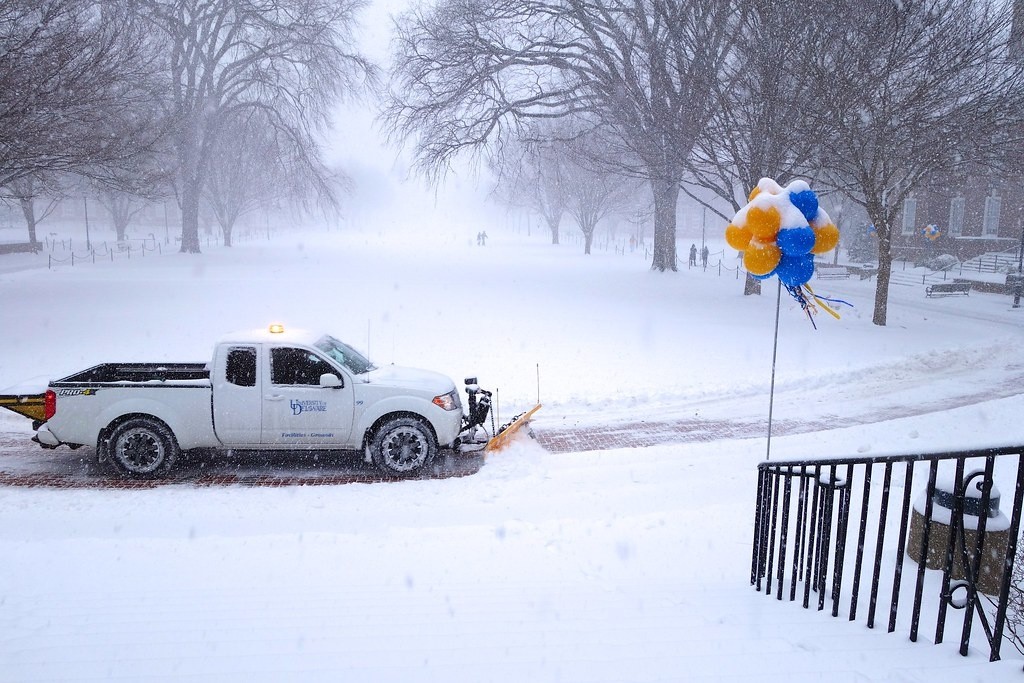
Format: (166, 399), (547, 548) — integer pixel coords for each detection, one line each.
(726, 180), (940, 328)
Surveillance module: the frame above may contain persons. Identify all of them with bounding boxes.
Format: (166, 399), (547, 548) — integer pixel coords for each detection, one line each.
(477, 230), (709, 267)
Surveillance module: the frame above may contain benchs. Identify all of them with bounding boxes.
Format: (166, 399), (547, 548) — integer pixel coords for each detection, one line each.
(925, 283), (972, 299)
(817, 267), (850, 281)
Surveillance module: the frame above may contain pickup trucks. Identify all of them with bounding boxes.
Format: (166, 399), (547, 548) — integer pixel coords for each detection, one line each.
(29, 322), (464, 477)
